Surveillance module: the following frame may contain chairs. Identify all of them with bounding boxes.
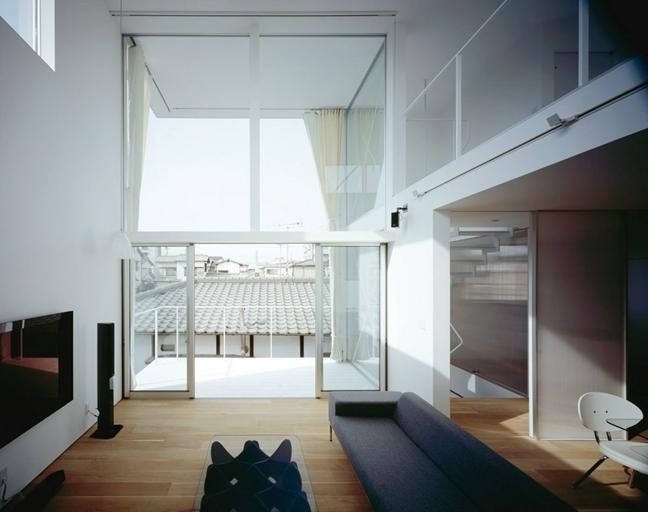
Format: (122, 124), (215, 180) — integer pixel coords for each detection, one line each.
(566, 393), (643, 490)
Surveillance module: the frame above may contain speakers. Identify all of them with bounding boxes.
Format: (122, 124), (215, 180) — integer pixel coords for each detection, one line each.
(90, 323), (122, 439)
(391, 213), (399, 227)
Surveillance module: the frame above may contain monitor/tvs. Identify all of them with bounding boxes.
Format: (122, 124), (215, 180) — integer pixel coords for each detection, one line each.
(0, 311), (74, 450)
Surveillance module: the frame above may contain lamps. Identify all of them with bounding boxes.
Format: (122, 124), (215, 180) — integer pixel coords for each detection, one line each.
(390, 203), (407, 228)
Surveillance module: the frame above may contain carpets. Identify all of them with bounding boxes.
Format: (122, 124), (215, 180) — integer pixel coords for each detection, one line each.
(193, 433), (318, 511)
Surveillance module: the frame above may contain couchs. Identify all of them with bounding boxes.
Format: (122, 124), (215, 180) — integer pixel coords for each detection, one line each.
(328, 389), (576, 511)
